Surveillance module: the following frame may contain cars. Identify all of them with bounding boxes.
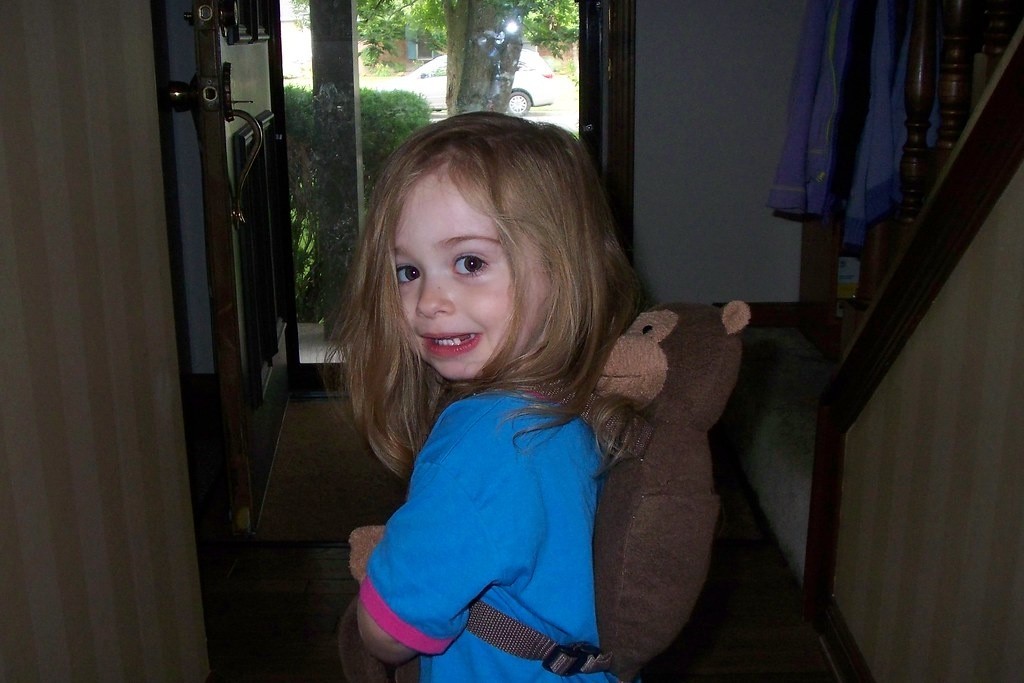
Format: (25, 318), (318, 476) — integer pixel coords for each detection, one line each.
(381, 49), (555, 116)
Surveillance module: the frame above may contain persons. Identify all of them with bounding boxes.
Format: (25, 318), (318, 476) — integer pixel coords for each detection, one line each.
(342, 114), (625, 682)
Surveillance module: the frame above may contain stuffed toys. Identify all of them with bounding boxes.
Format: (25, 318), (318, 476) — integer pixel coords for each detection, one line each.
(350, 301), (752, 677)
